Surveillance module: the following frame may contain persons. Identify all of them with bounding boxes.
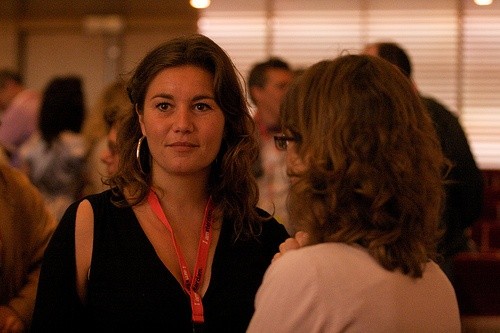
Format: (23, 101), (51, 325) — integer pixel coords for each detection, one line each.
(15, 72), (91, 195)
(245, 56), (292, 233)
(0, 69), (43, 159)
(245, 53), (460, 332)
(28, 33), (292, 333)
(82, 80), (133, 199)
(361, 41), (495, 244)
